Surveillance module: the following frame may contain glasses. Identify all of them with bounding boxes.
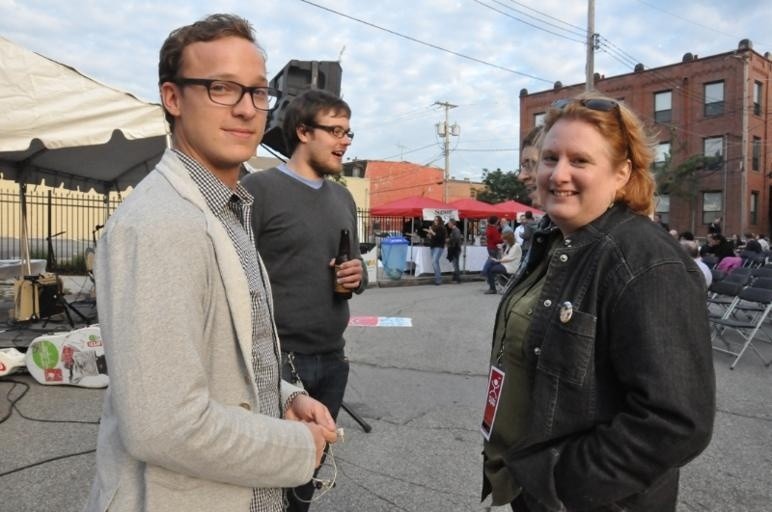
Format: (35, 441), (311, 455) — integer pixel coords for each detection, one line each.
(300, 120), (356, 144)
(157, 74), (283, 113)
(552, 95), (638, 163)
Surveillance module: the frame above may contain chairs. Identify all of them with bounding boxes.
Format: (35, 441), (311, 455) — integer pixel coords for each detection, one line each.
(696, 247), (772, 371)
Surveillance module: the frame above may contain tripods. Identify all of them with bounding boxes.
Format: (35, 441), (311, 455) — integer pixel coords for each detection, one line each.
(42, 240), (88, 328)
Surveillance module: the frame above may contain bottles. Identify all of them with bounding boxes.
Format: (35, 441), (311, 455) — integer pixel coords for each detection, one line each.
(332, 228), (355, 302)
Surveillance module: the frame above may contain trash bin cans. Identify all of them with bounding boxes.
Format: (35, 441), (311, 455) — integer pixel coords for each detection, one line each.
(381, 236), (409, 275)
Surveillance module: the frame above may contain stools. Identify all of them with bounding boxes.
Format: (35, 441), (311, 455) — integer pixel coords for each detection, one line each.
(497, 272), (517, 294)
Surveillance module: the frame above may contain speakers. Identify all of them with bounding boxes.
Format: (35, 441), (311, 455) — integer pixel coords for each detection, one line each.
(261, 59), (342, 159)
(14, 276), (62, 320)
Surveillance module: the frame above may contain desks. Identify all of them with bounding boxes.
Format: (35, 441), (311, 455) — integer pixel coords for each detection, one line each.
(406, 244), (487, 277)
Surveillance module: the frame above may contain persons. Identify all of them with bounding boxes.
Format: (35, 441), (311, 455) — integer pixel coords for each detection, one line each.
(517, 125), (545, 210)
(481, 91), (716, 512)
(82, 13), (336, 512)
(238, 87), (368, 512)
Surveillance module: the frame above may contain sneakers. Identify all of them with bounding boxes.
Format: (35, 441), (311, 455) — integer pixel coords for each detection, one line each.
(482, 289), (499, 295)
